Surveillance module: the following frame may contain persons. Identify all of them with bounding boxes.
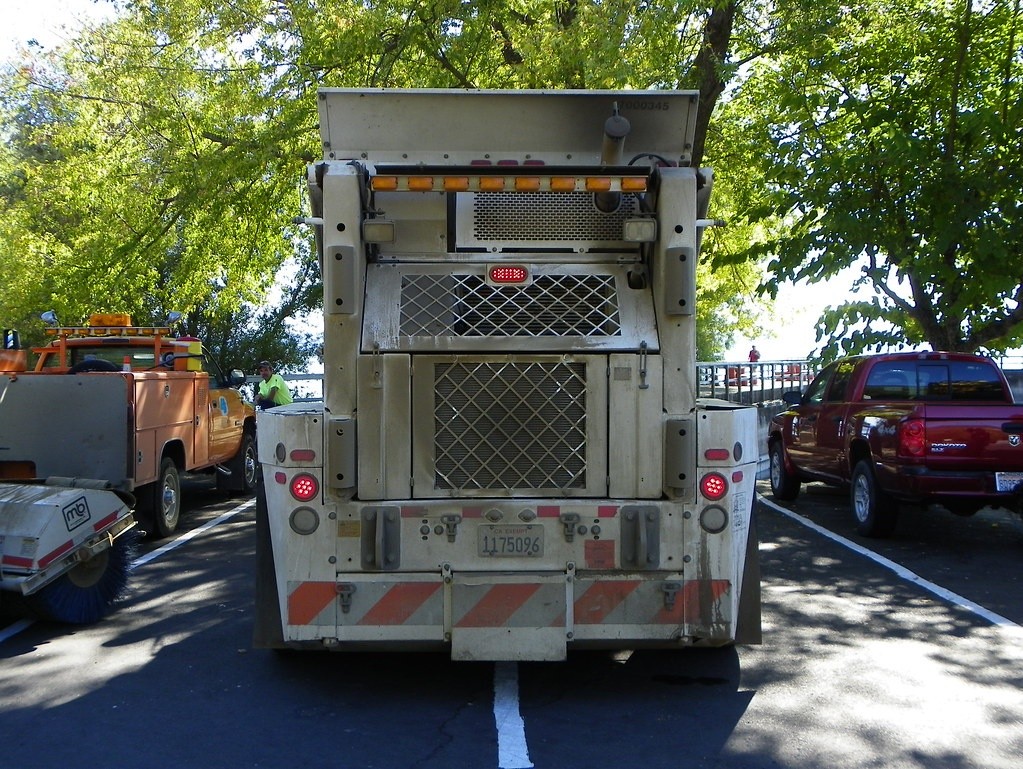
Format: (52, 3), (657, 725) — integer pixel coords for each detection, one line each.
(748, 345), (759, 372)
(254, 361), (293, 410)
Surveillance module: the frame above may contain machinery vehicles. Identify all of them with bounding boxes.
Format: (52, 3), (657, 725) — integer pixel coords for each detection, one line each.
(253, 84), (759, 660)
(0, 310), (258, 625)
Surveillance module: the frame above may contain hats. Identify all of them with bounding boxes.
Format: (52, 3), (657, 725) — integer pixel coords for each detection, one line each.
(257, 361), (273, 369)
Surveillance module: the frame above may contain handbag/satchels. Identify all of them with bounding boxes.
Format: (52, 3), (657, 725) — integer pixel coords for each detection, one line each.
(755, 350), (761, 359)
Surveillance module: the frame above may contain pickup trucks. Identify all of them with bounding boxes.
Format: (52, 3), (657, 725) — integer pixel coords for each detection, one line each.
(767, 351), (1022, 536)
